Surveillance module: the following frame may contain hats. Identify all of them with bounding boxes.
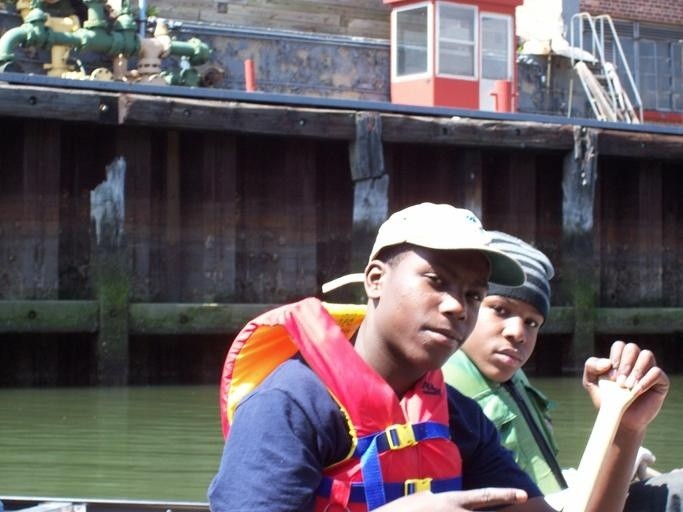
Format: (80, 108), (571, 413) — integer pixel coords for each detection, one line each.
(367, 202), (525, 289)
(484, 228), (555, 329)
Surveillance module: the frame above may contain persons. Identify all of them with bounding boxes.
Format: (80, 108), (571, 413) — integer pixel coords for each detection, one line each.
(440, 233), (682, 512)
(205, 203), (668, 511)
